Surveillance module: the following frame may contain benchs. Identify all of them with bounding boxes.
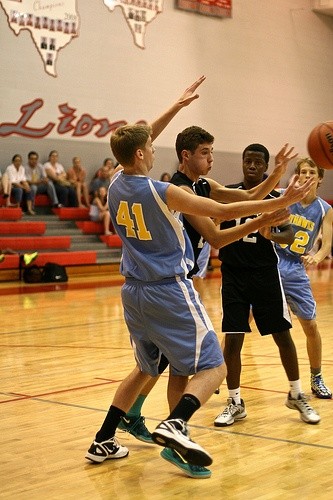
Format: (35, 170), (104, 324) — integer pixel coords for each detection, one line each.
(0, 193), (122, 276)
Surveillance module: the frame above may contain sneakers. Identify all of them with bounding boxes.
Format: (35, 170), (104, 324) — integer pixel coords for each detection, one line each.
(310, 373), (332, 401)
(213, 397), (248, 428)
(84, 435), (130, 463)
(160, 446), (212, 479)
(114, 414), (153, 444)
(285, 390), (321, 425)
(151, 419), (213, 467)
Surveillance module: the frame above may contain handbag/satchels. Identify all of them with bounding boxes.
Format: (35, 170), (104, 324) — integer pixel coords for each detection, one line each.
(23, 262), (69, 283)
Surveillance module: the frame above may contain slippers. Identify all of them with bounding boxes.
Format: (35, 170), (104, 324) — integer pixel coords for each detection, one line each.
(52, 203), (63, 208)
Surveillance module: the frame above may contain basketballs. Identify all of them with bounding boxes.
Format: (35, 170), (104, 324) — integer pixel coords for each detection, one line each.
(307, 121), (333, 170)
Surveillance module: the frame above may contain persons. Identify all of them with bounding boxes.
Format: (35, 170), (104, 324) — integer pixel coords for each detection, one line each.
(213, 157), (333, 400)
(213, 143), (323, 427)
(1, 150), (171, 236)
(115, 125), (301, 480)
(84, 74), (318, 468)
